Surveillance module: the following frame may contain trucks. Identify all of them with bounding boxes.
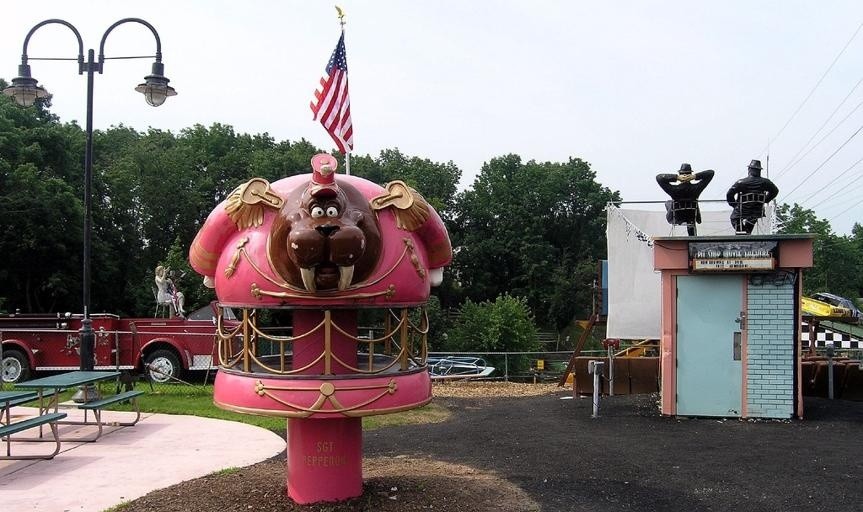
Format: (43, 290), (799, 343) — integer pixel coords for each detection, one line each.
(1, 300), (242, 384)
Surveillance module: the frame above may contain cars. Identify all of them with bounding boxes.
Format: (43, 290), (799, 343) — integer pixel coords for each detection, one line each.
(802, 291), (858, 320)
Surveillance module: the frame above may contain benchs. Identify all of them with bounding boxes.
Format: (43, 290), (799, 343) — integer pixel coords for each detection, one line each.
(0, 386), (145, 461)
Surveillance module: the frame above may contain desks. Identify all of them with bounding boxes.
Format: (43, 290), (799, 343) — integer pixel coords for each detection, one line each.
(14, 371), (120, 384)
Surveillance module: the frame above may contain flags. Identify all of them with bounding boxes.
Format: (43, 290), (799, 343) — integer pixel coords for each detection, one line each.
(309, 28), (354, 155)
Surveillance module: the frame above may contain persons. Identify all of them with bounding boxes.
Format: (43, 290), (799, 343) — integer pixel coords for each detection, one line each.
(727, 159), (779, 235)
(655, 163), (714, 237)
(154, 265), (186, 314)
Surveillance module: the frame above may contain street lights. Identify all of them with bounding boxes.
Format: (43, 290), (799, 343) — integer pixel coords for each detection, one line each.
(1, 17), (177, 403)
